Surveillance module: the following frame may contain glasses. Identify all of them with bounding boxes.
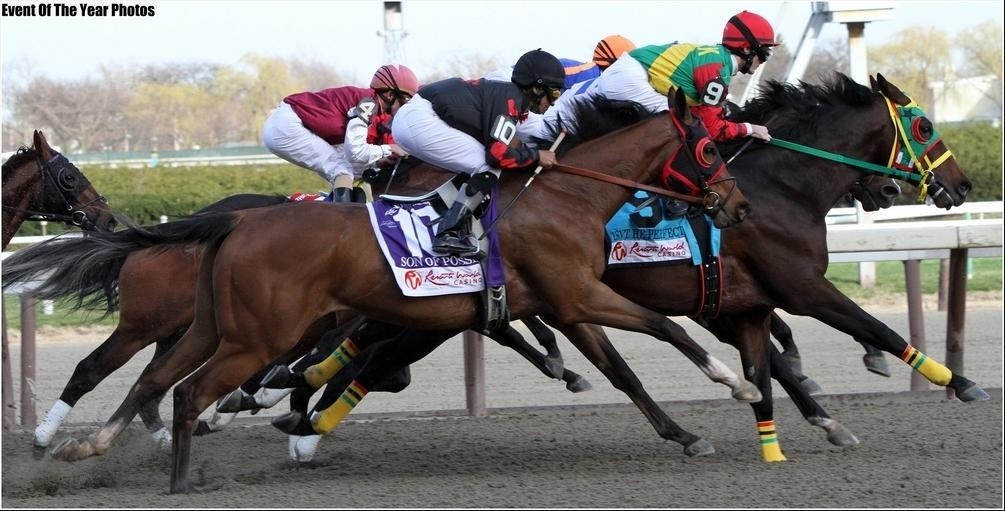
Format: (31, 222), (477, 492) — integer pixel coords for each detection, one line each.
(730, 16), (772, 63)
(375, 66), (413, 107)
(524, 55), (561, 106)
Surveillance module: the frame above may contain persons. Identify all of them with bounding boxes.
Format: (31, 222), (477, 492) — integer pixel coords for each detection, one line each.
(390, 48), (564, 260)
(483, 34), (642, 144)
(259, 64), (419, 200)
(583, 7), (779, 221)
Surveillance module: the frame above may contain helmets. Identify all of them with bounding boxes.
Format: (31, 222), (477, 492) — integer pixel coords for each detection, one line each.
(371, 64), (419, 95)
(593, 35), (634, 67)
(511, 49), (565, 88)
(722, 11), (780, 48)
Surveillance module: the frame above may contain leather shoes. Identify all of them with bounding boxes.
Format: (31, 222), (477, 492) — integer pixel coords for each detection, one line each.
(663, 197), (689, 216)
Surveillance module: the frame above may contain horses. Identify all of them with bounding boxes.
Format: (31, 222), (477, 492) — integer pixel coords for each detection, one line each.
(2, 129), (119, 255)
(2, 86), (764, 494)
(288, 169), (904, 468)
(471, 313), (824, 398)
(257, 71), (990, 464)
(1, 152), (456, 462)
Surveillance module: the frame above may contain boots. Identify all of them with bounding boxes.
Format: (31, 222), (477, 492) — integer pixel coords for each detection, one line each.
(432, 172), (497, 260)
